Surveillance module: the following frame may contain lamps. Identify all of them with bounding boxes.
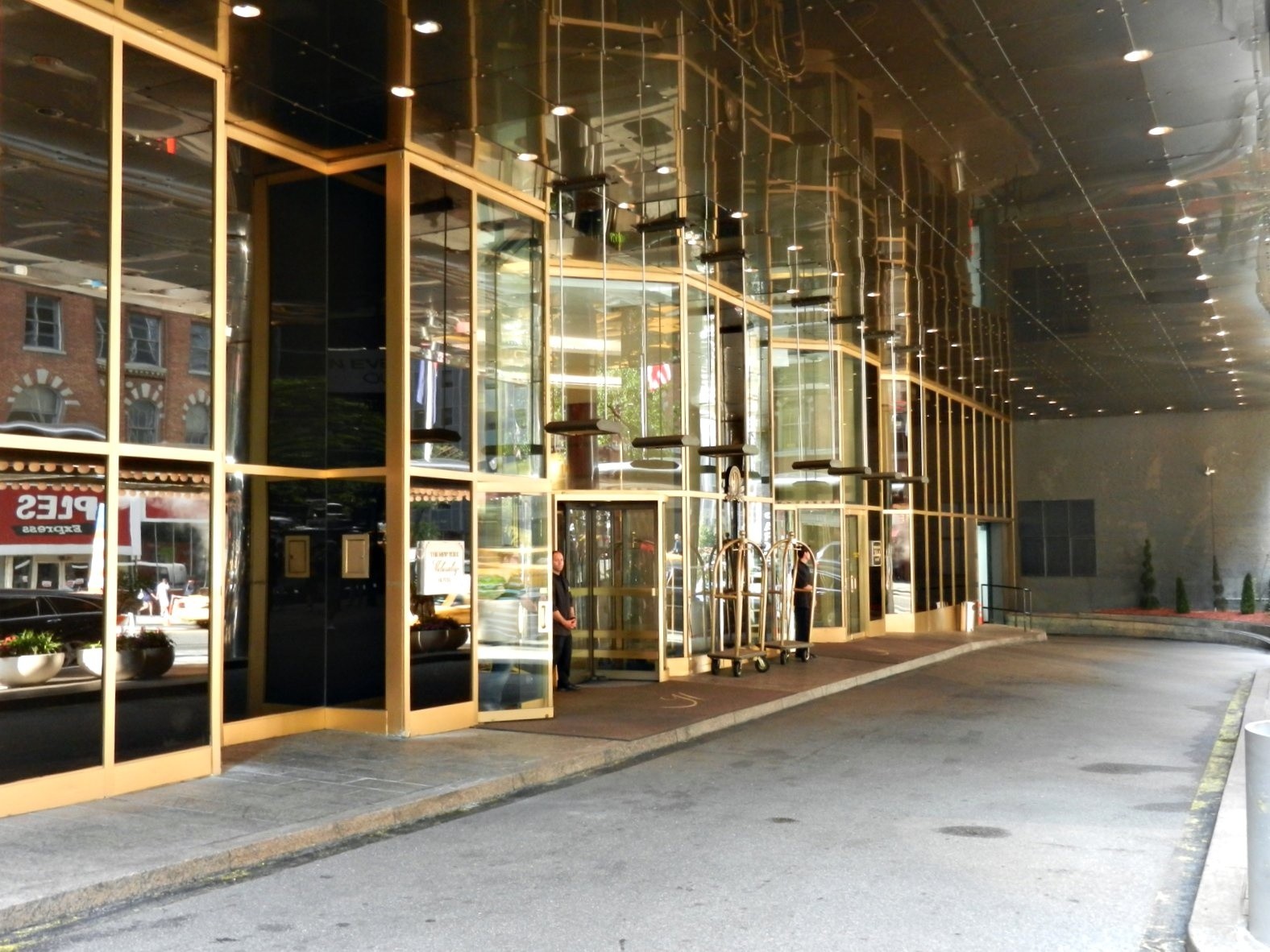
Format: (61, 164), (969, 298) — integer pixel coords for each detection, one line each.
(790, 294), (930, 484)
(544, 173), (623, 438)
(697, 245), (759, 458)
(632, 214), (700, 447)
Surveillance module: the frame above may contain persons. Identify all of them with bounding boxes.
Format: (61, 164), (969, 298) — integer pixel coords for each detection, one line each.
(553, 551), (581, 691)
(668, 533), (683, 577)
(479, 544), (546, 711)
(792, 545), (818, 658)
(183, 580), (196, 596)
(626, 548), (655, 670)
(137, 578), (170, 617)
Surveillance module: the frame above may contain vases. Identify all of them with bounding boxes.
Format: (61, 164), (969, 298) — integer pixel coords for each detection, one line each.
(76, 647), (175, 679)
(0, 653), (65, 688)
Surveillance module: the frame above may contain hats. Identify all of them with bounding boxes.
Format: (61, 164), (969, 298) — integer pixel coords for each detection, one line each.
(797, 545), (810, 552)
(674, 534), (681, 540)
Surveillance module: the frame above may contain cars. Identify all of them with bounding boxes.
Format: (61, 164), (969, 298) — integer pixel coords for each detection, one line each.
(696, 561), (762, 609)
(0, 589), (125, 668)
(788, 542), (841, 626)
(410, 547), (549, 644)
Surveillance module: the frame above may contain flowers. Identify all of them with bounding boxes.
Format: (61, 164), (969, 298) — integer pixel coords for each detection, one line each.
(81, 628), (175, 651)
(0, 631), (63, 657)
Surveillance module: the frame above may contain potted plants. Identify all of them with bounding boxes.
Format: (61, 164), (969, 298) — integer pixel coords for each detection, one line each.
(410, 617), (468, 653)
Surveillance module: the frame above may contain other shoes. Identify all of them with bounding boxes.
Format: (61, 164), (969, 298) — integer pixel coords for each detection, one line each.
(795, 652), (818, 658)
(557, 683), (580, 691)
(137, 609), (141, 615)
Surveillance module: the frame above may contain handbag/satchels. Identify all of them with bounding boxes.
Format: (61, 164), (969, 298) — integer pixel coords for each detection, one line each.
(136, 588), (143, 599)
